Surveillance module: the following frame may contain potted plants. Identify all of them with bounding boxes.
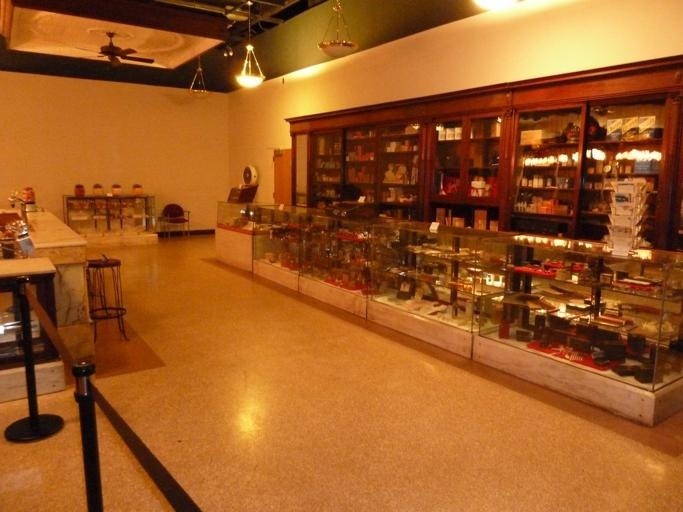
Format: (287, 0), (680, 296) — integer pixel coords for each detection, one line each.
(73, 183), (144, 198)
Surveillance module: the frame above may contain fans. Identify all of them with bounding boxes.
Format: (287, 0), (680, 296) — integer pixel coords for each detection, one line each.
(75, 32), (154, 68)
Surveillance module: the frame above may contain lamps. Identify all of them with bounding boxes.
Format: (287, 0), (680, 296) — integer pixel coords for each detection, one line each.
(235, 2), (266, 89)
(188, 54), (209, 99)
(317, 1), (358, 58)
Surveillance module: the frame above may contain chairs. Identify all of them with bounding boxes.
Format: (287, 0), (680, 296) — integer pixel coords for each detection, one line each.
(157, 204), (190, 241)
(86, 259), (131, 344)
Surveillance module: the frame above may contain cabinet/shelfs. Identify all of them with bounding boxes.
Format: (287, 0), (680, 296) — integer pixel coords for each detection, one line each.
(250, 203), (683, 428)
(286, 53), (682, 251)
(62, 194), (154, 235)
